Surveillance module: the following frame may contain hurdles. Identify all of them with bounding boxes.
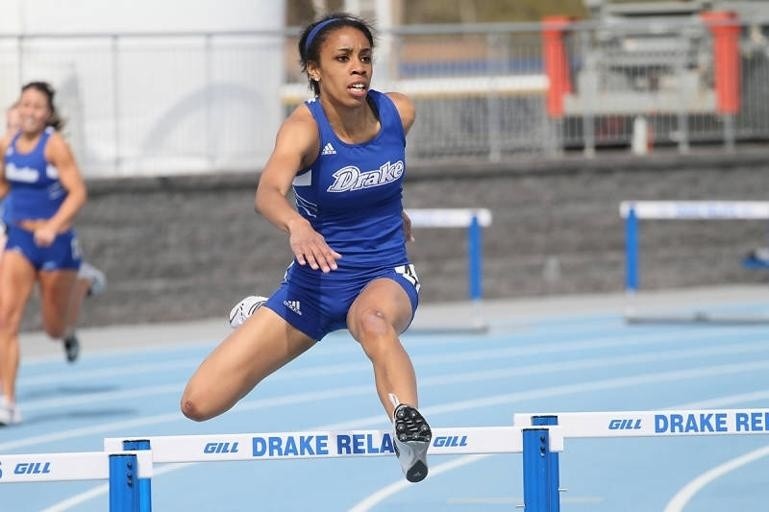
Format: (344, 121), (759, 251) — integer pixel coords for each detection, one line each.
(404, 207), (492, 303)
(515, 409), (769, 512)
(103, 426), (563, 512)
(0, 449), (155, 510)
(619, 202), (769, 293)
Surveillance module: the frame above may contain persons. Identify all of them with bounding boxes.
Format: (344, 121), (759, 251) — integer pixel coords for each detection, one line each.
(179, 9), (433, 484)
(3, 102), (106, 323)
(0, 81), (107, 427)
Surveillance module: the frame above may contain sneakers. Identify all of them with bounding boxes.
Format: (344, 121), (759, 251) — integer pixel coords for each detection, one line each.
(229, 294), (271, 330)
(392, 403), (434, 484)
(78, 261), (107, 298)
(0, 395), (22, 428)
(63, 333), (82, 363)
